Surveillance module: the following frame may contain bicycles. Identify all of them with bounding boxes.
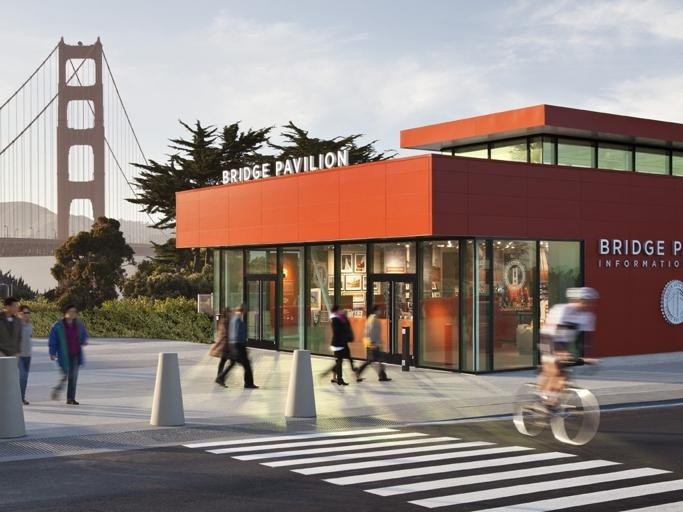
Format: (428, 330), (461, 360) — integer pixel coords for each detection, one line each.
(513, 362), (601, 446)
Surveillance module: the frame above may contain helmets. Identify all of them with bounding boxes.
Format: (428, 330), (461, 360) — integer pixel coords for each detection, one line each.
(567, 286), (599, 300)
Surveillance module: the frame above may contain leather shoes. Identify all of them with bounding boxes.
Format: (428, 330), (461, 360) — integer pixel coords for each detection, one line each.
(331, 377), (349, 385)
(357, 378), (392, 381)
(244, 384), (259, 388)
(215, 379), (228, 388)
(67, 400), (79, 405)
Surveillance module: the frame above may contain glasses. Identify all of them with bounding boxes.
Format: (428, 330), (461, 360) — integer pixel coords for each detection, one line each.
(23, 311), (31, 314)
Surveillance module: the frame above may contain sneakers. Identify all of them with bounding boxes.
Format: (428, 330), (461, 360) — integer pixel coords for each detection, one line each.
(21, 400), (28, 405)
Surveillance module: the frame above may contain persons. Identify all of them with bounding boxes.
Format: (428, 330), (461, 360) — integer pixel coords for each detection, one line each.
(48, 304), (89, 405)
(215, 303), (259, 389)
(16, 305), (33, 405)
(534, 287), (600, 413)
(356, 305), (392, 381)
(209, 307), (233, 377)
(0, 297), (22, 357)
(319, 304), (358, 385)
